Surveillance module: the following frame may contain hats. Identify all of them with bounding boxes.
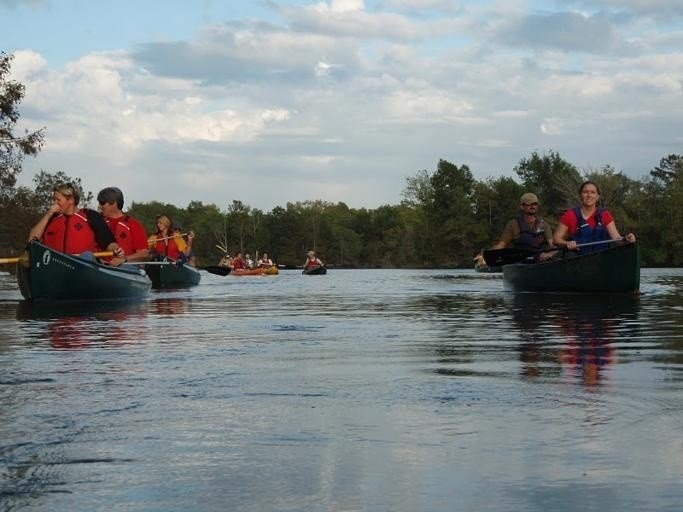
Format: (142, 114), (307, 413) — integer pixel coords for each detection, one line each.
(520, 193), (539, 205)
(156, 214), (172, 228)
(307, 251), (315, 255)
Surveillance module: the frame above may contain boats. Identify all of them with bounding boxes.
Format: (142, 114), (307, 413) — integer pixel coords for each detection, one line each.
(16, 239), (152, 303)
(196, 265), (279, 277)
(143, 253), (201, 289)
(302, 265), (327, 275)
(474, 263), (502, 273)
(502, 240), (641, 296)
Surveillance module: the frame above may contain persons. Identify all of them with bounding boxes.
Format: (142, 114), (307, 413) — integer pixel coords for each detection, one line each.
(491, 193), (553, 265)
(551, 180), (636, 261)
(219, 252), (273, 270)
(27, 180), (128, 267)
(302, 250), (324, 268)
(472, 248), (486, 267)
(146, 213), (195, 263)
(90, 187), (150, 272)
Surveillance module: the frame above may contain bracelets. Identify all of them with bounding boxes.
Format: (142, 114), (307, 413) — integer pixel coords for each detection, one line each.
(122, 256), (127, 264)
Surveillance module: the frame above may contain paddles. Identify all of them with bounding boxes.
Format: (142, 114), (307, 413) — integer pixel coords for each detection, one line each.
(195, 265), (231, 276)
(482, 237), (625, 267)
